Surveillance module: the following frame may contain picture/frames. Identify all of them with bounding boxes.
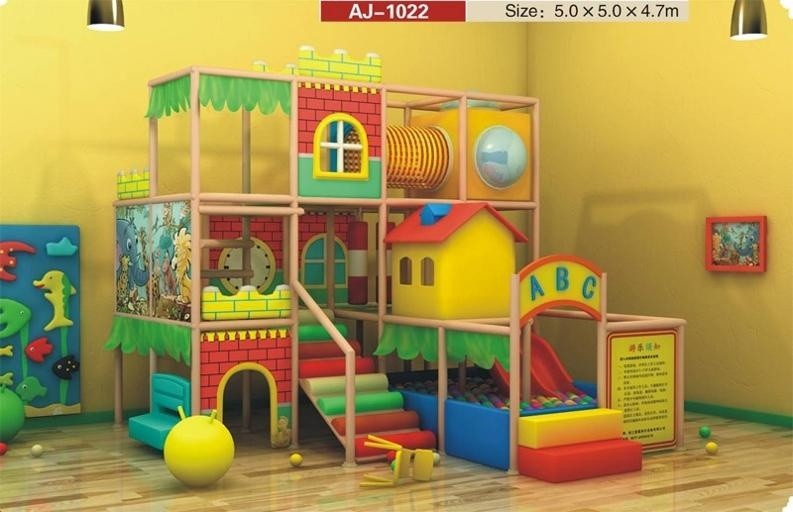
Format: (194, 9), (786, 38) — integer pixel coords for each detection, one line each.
(705, 214), (765, 273)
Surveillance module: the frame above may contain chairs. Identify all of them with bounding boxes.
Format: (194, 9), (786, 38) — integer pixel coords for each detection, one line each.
(358, 432), (437, 486)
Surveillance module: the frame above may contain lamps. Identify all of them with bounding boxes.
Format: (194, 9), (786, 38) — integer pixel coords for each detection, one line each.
(85, 0), (124, 34)
(728, 0), (770, 43)
